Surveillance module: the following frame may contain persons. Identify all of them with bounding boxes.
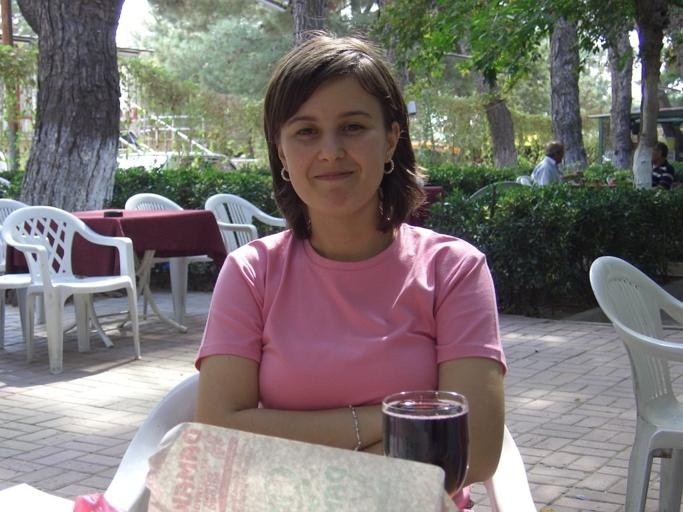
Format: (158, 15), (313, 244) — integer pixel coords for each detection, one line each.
(652, 143), (675, 190)
(531, 141), (564, 184)
(194, 31), (507, 512)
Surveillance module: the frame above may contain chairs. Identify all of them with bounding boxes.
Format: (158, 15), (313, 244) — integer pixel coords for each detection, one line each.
(104, 370), (537, 512)
(587, 254), (682, 511)
(0, 191), (287, 375)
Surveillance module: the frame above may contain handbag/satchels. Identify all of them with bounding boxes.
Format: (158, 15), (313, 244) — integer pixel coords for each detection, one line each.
(349, 405), (362, 452)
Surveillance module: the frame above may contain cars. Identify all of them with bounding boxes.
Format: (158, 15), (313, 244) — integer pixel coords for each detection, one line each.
(380, 388), (471, 500)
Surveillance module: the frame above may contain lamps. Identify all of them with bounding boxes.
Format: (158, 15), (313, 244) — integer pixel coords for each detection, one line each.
(104, 370), (537, 512)
(587, 254), (682, 511)
